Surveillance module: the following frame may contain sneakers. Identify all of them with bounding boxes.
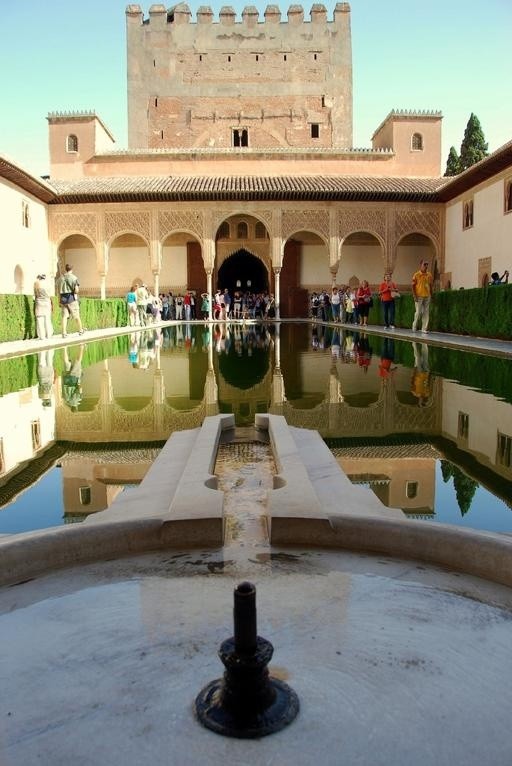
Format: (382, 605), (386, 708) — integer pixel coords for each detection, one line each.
(313, 317), (368, 327)
(79, 328), (87, 335)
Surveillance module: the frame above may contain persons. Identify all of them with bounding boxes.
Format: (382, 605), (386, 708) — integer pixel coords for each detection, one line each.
(60, 344), (85, 411)
(411, 339), (434, 407)
(128, 325), (275, 369)
(311, 278), (372, 324)
(127, 281), (275, 326)
(312, 324), (371, 371)
(377, 337), (397, 386)
(410, 258), (434, 334)
(36, 347), (55, 408)
(378, 272), (401, 330)
(32, 272), (58, 339)
(489, 268), (510, 286)
(56, 264), (89, 338)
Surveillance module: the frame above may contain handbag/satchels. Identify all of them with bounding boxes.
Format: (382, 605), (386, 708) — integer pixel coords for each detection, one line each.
(60, 293), (75, 304)
(391, 291), (401, 298)
